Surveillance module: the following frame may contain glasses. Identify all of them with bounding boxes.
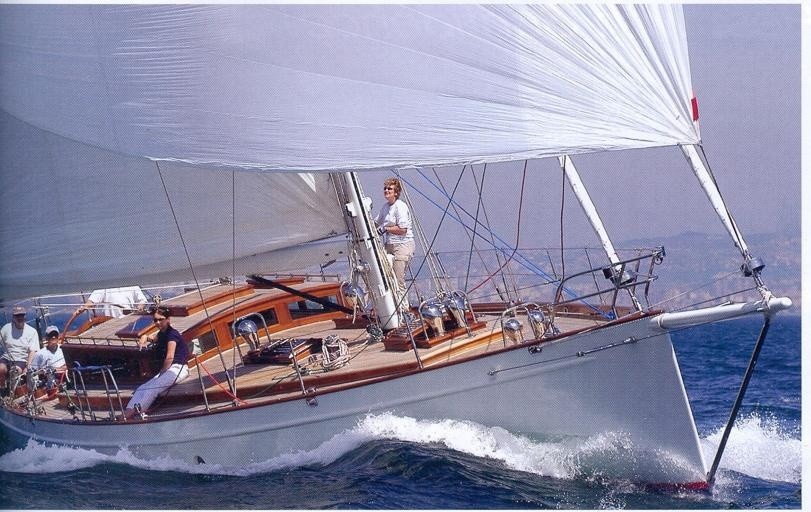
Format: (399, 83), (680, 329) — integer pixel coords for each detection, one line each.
(154, 317), (166, 323)
(12, 307), (27, 316)
(383, 187), (394, 191)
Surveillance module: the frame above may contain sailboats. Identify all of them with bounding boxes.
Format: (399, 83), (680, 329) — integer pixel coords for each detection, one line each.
(1, 4), (792, 495)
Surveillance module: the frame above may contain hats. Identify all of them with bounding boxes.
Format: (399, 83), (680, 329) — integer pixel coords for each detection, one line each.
(45, 325), (59, 335)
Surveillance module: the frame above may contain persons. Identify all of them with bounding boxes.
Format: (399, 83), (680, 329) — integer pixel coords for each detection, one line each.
(73, 286), (148, 318)
(114, 307), (191, 421)
(373, 178), (416, 323)
(31, 326), (66, 371)
(0, 307), (40, 398)
